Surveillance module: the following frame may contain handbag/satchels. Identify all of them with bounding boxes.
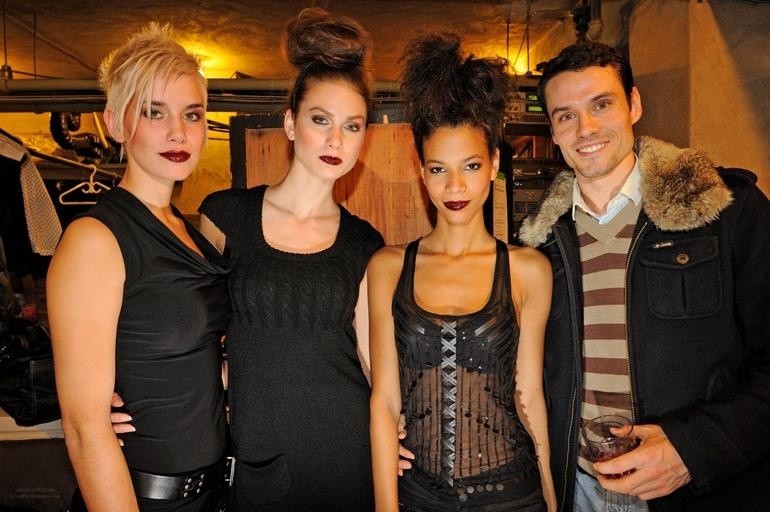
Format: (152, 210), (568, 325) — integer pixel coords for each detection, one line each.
(0, 321), (62, 427)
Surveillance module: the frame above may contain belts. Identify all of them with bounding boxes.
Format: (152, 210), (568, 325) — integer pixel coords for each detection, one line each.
(129, 453), (238, 502)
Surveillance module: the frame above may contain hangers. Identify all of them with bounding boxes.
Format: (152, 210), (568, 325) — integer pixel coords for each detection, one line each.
(60, 163), (112, 205)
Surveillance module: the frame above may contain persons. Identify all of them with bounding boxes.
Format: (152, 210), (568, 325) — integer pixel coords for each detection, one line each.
(46, 23), (234, 512)
(365, 28), (561, 512)
(101, 7), (386, 512)
(396, 42), (770, 512)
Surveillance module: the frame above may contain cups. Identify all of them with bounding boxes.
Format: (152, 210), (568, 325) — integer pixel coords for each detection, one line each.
(580, 412), (635, 483)
(12, 287), (37, 322)
